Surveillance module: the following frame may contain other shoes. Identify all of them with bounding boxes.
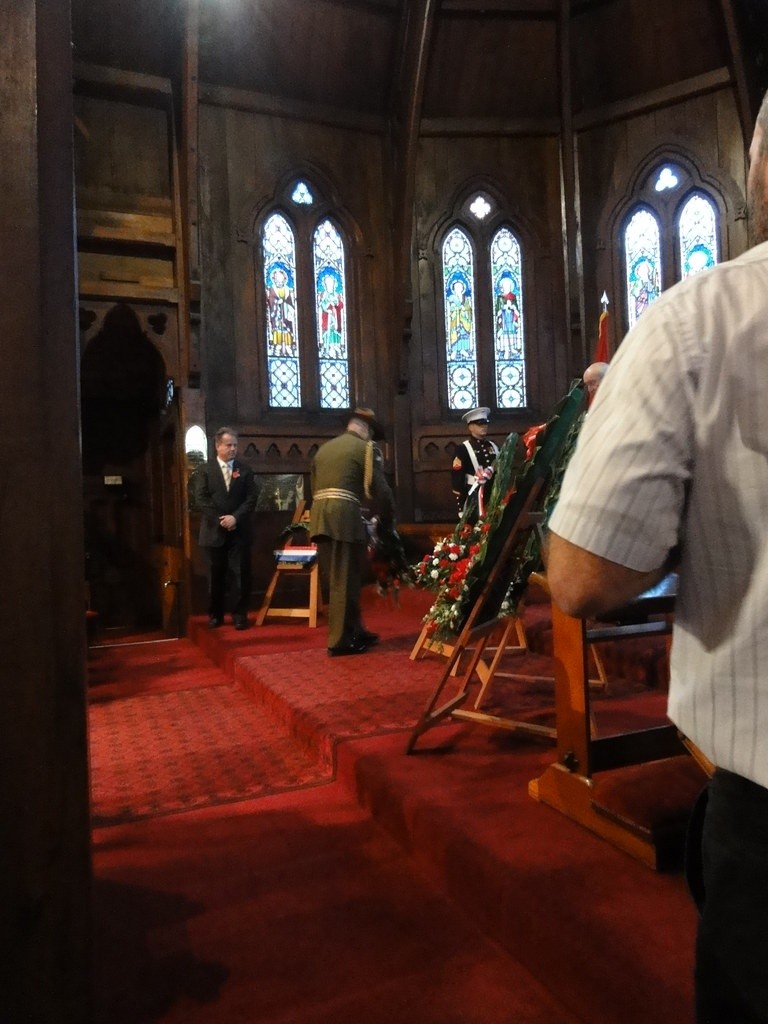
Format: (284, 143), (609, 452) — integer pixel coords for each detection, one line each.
(232, 614), (249, 630)
(208, 614), (225, 627)
(327, 631), (379, 657)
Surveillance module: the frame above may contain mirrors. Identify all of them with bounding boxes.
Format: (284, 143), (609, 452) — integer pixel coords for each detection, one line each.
(185, 425), (209, 513)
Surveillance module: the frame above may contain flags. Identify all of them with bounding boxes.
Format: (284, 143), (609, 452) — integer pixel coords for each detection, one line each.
(588, 309), (610, 408)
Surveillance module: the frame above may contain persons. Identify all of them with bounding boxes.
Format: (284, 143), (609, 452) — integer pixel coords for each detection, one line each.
(193, 427), (261, 630)
(542, 90), (768, 1023)
(449, 407), (499, 526)
(308, 406), (401, 656)
(583, 361), (609, 395)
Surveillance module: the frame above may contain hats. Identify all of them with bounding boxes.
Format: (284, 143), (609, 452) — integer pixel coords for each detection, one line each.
(462, 407), (491, 424)
(351, 407), (376, 427)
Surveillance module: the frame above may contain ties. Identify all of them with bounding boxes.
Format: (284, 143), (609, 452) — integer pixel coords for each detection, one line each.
(224, 464), (231, 493)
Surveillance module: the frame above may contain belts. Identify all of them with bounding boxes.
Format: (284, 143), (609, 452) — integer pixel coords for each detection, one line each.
(313, 487), (362, 505)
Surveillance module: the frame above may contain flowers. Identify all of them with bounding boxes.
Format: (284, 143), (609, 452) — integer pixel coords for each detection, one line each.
(367, 424), (546, 659)
(233, 471), (239, 478)
